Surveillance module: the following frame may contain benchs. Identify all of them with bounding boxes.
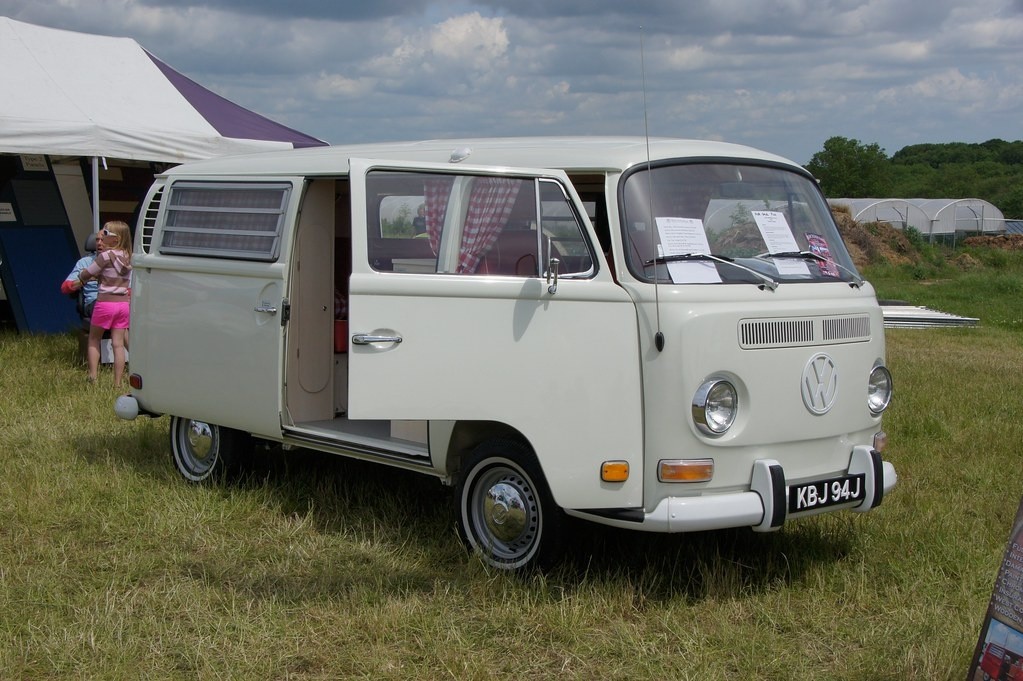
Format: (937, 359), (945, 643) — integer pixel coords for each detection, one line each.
(335, 230), (437, 278)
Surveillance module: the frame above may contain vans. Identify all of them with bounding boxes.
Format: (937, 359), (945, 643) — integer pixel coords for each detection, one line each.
(115, 137), (898, 580)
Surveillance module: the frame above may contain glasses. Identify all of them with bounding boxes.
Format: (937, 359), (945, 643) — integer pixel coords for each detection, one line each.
(103, 228), (118, 237)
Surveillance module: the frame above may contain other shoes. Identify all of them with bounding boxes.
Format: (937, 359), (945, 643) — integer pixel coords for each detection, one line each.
(87, 374), (99, 387)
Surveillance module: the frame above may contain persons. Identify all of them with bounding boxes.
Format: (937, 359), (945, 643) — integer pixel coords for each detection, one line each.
(61, 230), (129, 351)
(81, 220), (133, 386)
(410, 207), (426, 238)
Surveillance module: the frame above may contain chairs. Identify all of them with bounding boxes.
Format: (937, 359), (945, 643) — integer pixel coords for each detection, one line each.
(79, 232), (129, 364)
(476, 187), (568, 275)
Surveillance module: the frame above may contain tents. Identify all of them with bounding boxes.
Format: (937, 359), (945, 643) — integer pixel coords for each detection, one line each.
(0, 16), (331, 232)
